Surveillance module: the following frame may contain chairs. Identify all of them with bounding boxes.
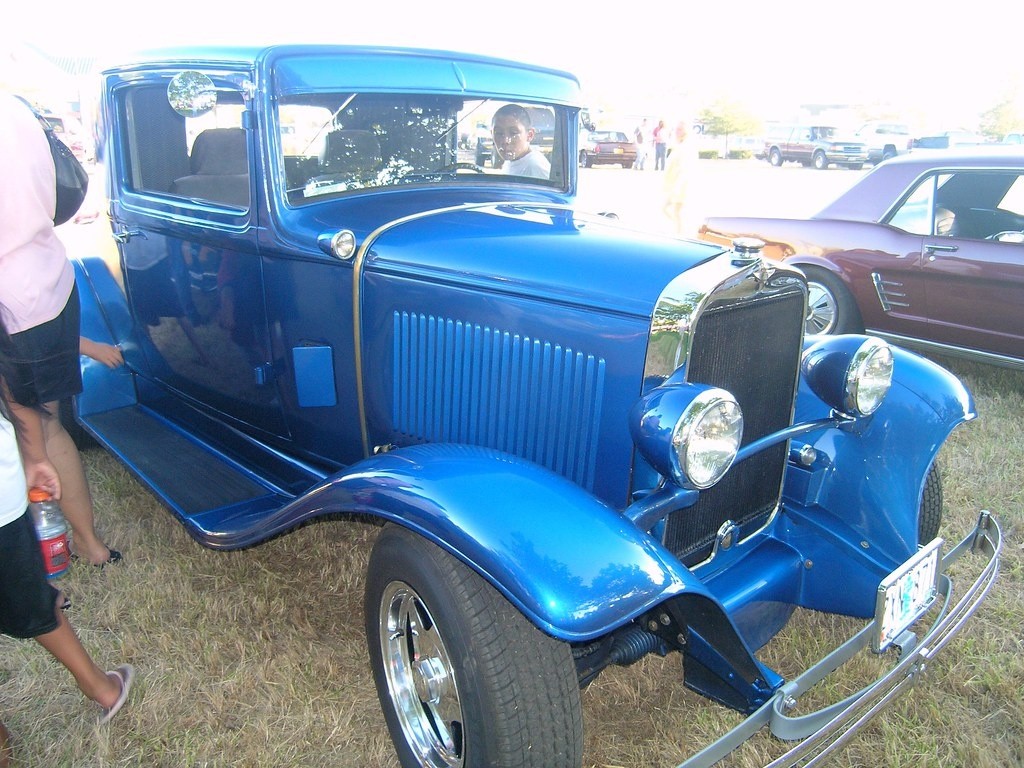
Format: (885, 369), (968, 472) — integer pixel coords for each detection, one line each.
(166, 127), (249, 206)
(320, 130), (382, 182)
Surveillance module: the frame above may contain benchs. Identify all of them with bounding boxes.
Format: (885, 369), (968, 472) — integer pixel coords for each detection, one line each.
(935, 199), (982, 238)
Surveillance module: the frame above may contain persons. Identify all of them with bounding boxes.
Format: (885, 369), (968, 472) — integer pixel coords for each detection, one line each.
(0, 91), (136, 768)
(634, 117), (694, 233)
(492, 103), (551, 179)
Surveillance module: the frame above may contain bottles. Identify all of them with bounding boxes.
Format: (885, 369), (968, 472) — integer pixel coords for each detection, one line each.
(28, 487), (71, 579)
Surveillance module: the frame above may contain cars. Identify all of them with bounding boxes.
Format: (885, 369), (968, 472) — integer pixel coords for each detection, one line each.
(695, 145), (1024, 372)
(473, 106), (556, 170)
(851, 121), (949, 166)
(578, 130), (638, 170)
(64, 43), (1002, 768)
(762, 123), (869, 171)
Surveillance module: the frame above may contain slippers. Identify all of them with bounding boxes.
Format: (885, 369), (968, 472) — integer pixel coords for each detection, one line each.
(64, 538), (123, 569)
(93, 662), (136, 726)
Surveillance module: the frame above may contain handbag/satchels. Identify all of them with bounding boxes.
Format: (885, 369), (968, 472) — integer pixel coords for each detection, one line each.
(13, 93), (89, 226)
